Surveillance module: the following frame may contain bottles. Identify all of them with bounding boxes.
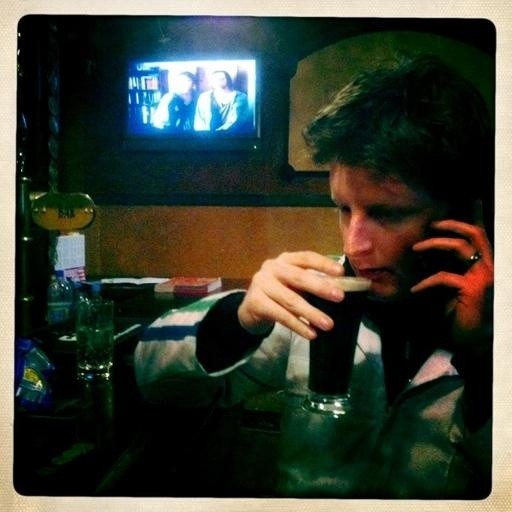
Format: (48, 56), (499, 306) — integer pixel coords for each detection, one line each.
(45, 270), (75, 345)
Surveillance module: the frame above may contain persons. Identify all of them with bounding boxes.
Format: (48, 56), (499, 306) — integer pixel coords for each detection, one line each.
(149, 71), (203, 132)
(192, 71), (249, 134)
(131, 50), (496, 498)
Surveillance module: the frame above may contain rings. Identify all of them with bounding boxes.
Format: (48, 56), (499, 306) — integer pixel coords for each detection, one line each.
(464, 251), (480, 268)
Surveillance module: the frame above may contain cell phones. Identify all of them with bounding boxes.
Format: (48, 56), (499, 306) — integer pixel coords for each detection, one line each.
(401, 195), (478, 281)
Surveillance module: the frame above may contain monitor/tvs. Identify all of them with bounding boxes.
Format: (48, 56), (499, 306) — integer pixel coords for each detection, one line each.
(119, 50), (265, 153)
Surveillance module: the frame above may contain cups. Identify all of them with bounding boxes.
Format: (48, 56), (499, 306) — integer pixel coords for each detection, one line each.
(75, 298), (115, 382)
(302, 276), (373, 417)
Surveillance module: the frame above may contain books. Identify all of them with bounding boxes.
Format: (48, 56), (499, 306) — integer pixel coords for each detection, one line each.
(127, 76), (162, 128)
(153, 275), (222, 294)
